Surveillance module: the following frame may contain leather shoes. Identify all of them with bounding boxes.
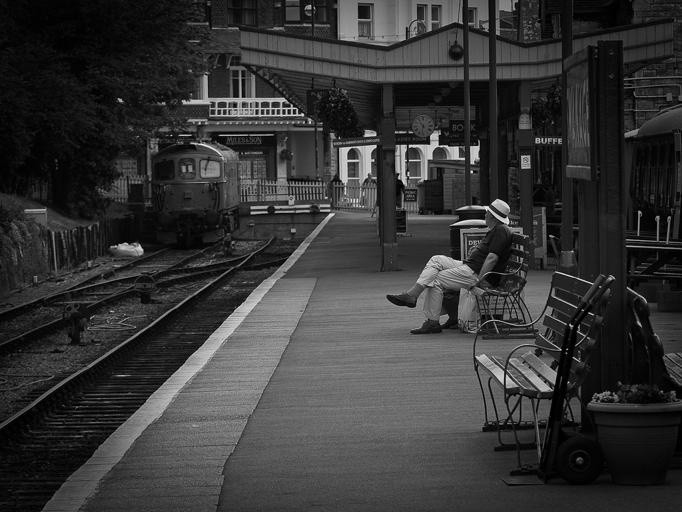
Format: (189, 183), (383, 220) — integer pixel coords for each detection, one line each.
(386, 292), (417, 308)
(410, 320), (442, 335)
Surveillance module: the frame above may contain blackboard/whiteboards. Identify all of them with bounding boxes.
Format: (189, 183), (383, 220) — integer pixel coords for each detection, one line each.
(533, 207), (547, 259)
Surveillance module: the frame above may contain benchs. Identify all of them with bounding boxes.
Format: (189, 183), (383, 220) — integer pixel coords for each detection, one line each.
(473, 271), (604, 473)
(467, 234), (539, 339)
(619, 279), (680, 398)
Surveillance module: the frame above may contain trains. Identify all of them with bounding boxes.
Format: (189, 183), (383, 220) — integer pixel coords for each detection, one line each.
(151, 122), (242, 249)
(624, 104), (682, 234)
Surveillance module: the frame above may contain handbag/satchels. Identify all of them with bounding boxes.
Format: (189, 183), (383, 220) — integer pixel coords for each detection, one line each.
(457, 285), (483, 334)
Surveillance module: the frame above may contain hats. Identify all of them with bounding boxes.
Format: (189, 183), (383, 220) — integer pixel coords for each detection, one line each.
(483, 197), (511, 225)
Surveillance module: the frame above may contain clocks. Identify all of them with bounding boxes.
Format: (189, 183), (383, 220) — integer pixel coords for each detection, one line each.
(411, 113), (435, 138)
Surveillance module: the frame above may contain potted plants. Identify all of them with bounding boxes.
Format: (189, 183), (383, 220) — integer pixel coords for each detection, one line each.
(585, 381), (679, 476)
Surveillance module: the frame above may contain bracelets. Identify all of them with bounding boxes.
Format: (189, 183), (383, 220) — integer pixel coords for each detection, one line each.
(474, 277), (480, 282)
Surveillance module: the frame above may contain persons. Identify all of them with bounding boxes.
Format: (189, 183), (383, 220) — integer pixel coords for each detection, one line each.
(385, 198), (514, 335)
(329, 174), (343, 201)
(531, 170), (557, 252)
(362, 171), (377, 188)
(313, 176), (320, 185)
(396, 170), (406, 208)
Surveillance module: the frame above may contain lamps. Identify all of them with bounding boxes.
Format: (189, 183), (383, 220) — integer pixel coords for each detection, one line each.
(427, 82), (456, 109)
